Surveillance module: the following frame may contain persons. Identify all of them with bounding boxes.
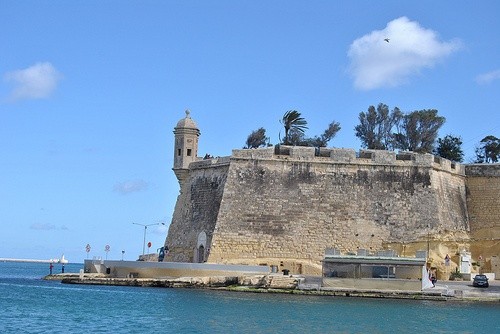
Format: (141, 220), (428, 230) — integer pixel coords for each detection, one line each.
(328, 269), (334, 278)
(333, 270), (337, 278)
(432, 274), (437, 287)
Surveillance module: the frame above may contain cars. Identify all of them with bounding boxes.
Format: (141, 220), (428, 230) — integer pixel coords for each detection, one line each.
(473, 274), (489, 288)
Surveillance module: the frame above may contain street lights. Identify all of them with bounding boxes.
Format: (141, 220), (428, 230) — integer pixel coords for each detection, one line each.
(131, 222), (164, 255)
